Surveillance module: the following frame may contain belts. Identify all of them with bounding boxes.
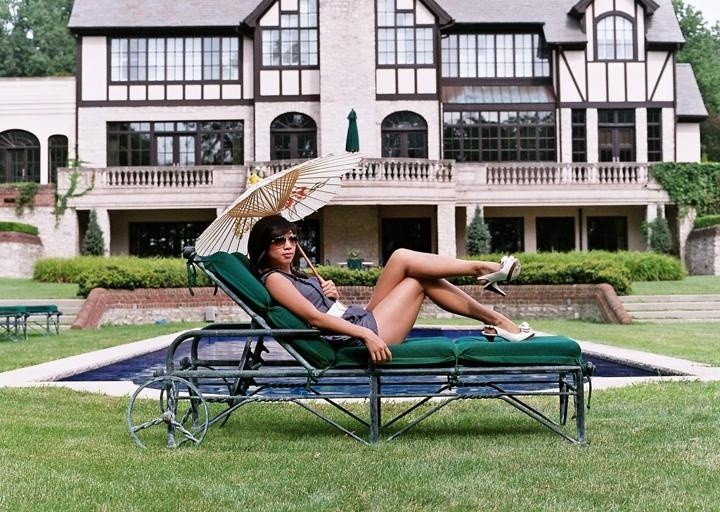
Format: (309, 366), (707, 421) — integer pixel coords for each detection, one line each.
(326, 298), (349, 318)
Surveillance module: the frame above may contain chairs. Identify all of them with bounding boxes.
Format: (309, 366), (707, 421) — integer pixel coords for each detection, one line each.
(127, 247), (597, 450)
(0, 304), (62, 341)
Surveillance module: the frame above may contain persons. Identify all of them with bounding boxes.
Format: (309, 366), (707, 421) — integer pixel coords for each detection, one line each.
(248, 215), (536, 366)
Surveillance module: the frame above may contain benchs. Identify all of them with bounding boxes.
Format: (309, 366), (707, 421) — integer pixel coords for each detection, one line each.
(335, 261), (373, 271)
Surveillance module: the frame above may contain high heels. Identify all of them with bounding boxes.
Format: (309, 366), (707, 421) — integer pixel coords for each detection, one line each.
(481, 324), (535, 342)
(477, 256), (520, 295)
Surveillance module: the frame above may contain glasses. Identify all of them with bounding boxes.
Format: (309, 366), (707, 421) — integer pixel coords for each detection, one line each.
(268, 235), (298, 246)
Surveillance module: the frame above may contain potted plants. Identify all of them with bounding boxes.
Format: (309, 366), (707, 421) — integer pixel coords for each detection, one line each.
(345, 248), (363, 270)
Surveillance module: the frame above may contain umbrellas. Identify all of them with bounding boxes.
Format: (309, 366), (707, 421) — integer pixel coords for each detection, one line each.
(191, 149), (368, 285)
(344, 107), (360, 152)
